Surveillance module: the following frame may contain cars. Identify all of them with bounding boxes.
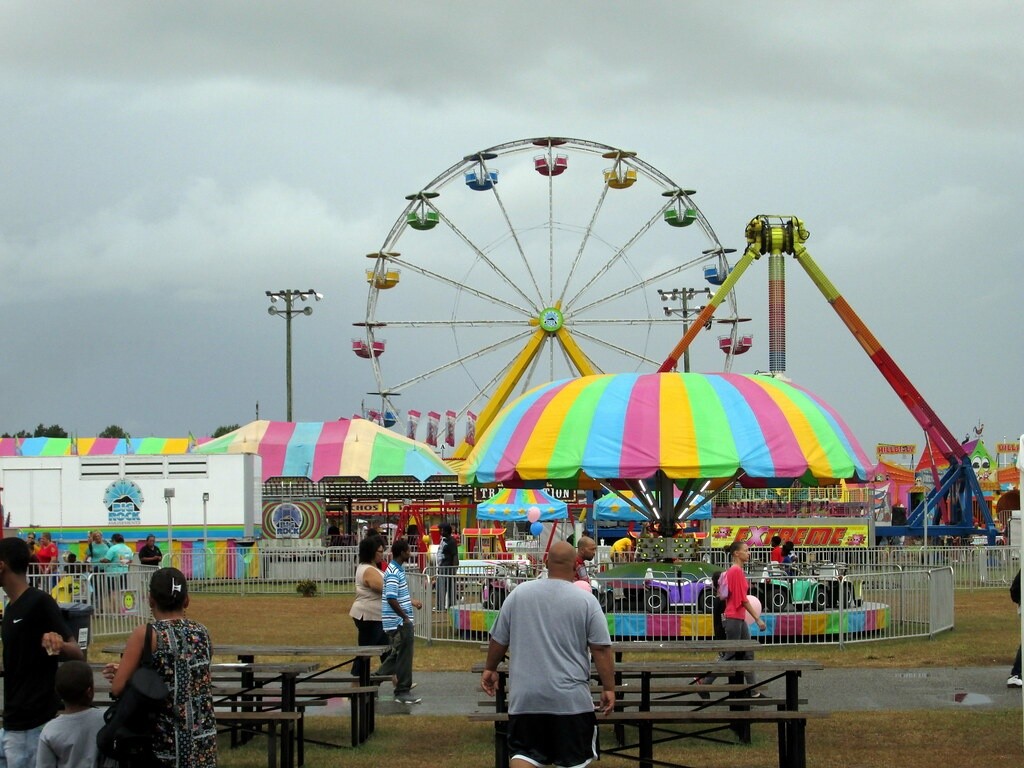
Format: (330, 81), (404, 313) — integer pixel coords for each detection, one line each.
(479, 563), (616, 614)
(750, 560), (865, 612)
(644, 566), (715, 614)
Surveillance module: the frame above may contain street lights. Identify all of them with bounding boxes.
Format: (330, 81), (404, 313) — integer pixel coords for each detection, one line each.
(163, 488), (176, 555)
(202, 493), (210, 550)
(264, 287), (323, 422)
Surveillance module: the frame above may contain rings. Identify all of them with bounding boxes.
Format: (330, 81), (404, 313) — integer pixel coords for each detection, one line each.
(418, 604), (421, 606)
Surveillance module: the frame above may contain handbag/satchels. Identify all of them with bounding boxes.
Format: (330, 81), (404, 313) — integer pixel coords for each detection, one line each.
(96, 623), (170, 761)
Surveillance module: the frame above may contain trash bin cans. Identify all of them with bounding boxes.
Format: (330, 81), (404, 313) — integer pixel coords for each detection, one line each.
(61, 602), (94, 660)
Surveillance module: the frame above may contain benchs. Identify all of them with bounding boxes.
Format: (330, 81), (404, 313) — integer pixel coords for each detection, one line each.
(468, 672), (832, 768)
(59, 663), (399, 768)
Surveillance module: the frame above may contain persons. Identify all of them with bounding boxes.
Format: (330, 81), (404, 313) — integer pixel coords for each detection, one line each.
(326, 519), (388, 545)
(432, 522), (464, 613)
(769, 536), (800, 584)
(370, 539), (421, 704)
(24, 530), (163, 593)
(348, 535), (423, 692)
(1006, 568), (1022, 688)
(35, 660), (114, 768)
(695, 539), (766, 700)
(536, 529), (636, 594)
(481, 541), (615, 768)
(0, 537), (84, 768)
(104, 567), (218, 768)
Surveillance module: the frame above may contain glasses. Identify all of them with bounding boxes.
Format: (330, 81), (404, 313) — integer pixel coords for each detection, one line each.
(377, 550), (385, 553)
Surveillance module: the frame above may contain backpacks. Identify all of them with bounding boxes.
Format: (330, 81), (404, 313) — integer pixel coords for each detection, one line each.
(716, 571), (729, 600)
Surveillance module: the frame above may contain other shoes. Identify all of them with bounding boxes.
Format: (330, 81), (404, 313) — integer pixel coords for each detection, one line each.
(752, 693), (772, 699)
(1007, 675), (1023, 687)
(393, 683), (418, 692)
(371, 672), (382, 700)
(456, 596), (466, 605)
(394, 695), (421, 703)
(432, 606), (447, 612)
(694, 676), (711, 699)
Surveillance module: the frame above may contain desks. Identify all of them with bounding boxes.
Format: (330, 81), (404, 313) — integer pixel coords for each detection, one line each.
(102, 645), (393, 743)
(480, 639), (763, 744)
(89, 664), (320, 768)
(472, 660), (825, 768)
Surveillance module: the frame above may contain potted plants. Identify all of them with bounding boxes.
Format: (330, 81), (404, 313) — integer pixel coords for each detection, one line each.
(295, 579), (317, 598)
